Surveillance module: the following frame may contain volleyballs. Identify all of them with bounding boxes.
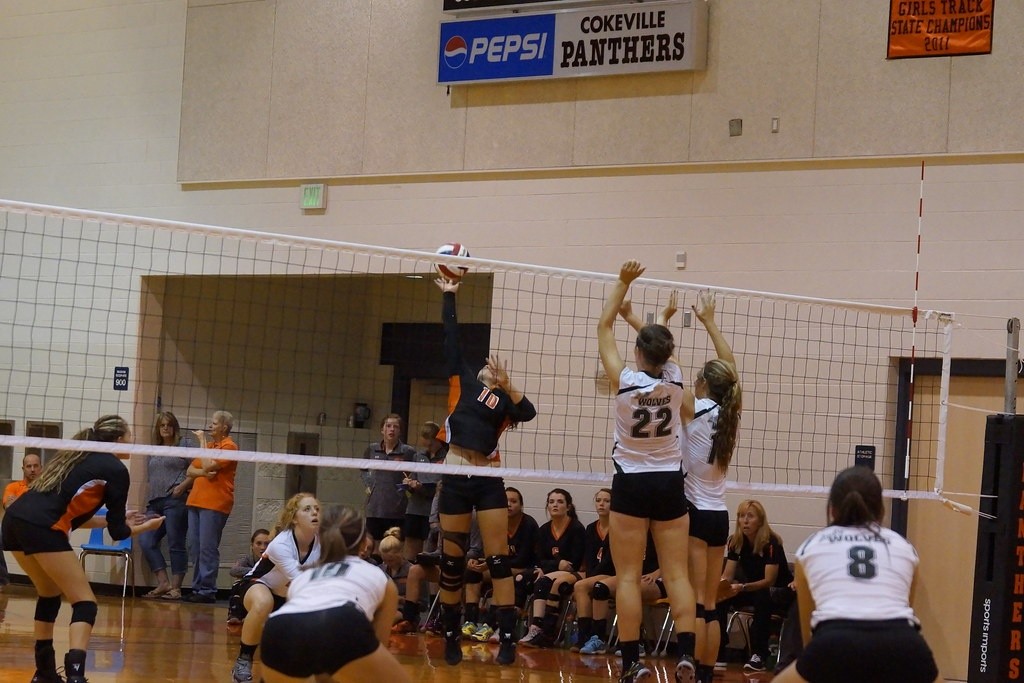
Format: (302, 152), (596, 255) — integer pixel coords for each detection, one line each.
(433, 240), (471, 282)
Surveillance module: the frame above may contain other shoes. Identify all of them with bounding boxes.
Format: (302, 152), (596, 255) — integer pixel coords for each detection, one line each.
(189, 593), (216, 602)
(444, 622), (463, 665)
(496, 625), (516, 663)
(183, 593), (197, 601)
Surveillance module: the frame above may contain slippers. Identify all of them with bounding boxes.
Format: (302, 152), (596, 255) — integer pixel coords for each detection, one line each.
(142, 588), (163, 597)
(162, 588), (181, 599)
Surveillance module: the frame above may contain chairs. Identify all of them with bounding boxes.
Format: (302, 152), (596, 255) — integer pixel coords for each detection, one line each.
(84, 599), (134, 674)
(419, 554), (802, 675)
(78, 508), (136, 598)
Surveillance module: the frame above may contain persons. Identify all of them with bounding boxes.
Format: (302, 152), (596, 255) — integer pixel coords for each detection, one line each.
(227, 529), (271, 624)
(139, 410), (238, 603)
(770, 466), (938, 683)
(260, 505), (409, 683)
(348, 413), (793, 671)
(434, 277), (537, 666)
(2, 413), (166, 683)
(2, 454), (42, 517)
(230, 492), (346, 683)
(597, 260), (741, 683)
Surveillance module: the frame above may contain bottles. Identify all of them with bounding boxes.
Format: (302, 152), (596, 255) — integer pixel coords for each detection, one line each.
(348, 413), (354, 427)
(571, 622), (579, 645)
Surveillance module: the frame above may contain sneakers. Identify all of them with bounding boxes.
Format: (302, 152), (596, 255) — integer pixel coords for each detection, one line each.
(461, 621), (499, 641)
(232, 656), (252, 683)
(619, 662), (650, 683)
(227, 624), (241, 635)
(743, 654), (765, 671)
(227, 602), (241, 623)
(31, 665), (67, 683)
(675, 655), (695, 683)
(67, 676), (90, 683)
(744, 671), (765, 679)
(714, 649), (728, 667)
(518, 623), (645, 656)
(459, 643), (498, 662)
(392, 617), (442, 635)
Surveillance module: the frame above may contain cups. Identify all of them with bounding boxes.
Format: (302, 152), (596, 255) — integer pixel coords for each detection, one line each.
(354, 402), (370, 428)
